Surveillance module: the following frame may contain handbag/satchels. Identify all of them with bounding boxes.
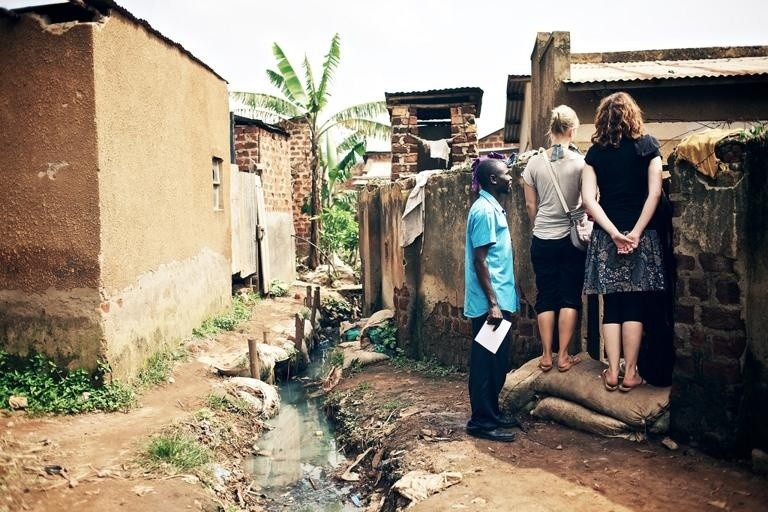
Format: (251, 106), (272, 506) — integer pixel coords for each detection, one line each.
(646, 187), (673, 229)
(570, 220), (594, 248)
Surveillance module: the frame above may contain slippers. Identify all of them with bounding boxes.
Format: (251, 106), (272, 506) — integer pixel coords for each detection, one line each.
(601, 363), (646, 390)
(558, 356), (581, 371)
(541, 365), (552, 370)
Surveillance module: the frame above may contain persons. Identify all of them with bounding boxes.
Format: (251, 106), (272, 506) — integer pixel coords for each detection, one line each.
(520, 103), (599, 373)
(463, 157), (522, 443)
(580, 90), (671, 392)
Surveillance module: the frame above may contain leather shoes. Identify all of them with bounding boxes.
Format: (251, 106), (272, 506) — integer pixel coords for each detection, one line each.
(468, 428), (515, 441)
(495, 412), (519, 426)
